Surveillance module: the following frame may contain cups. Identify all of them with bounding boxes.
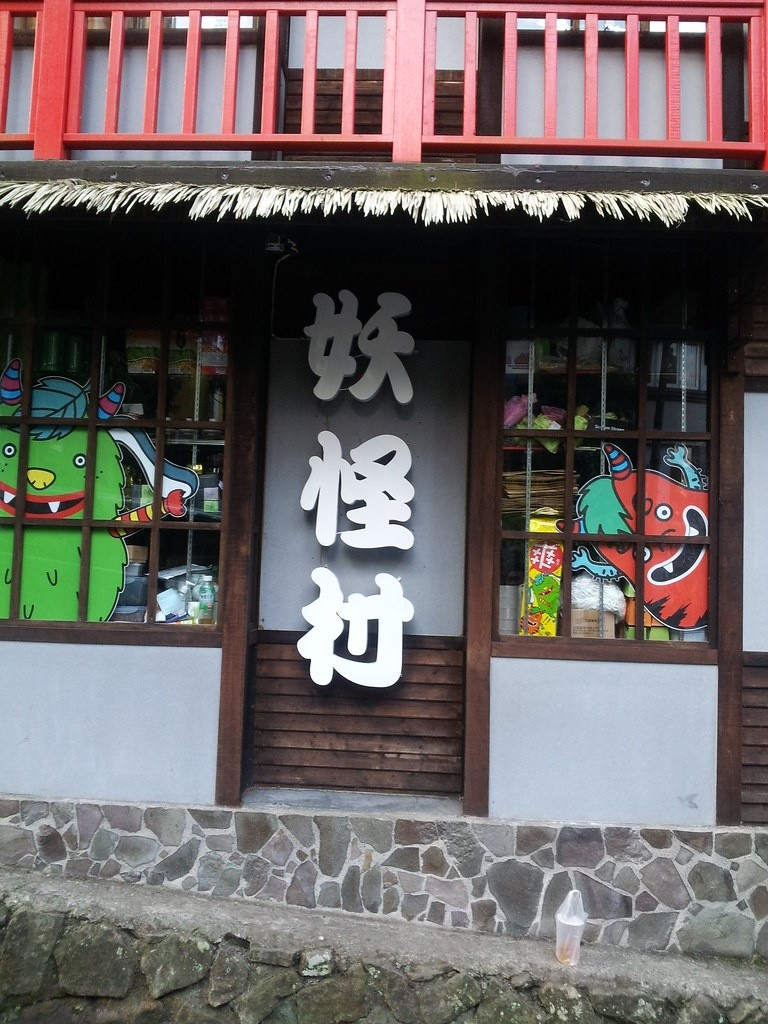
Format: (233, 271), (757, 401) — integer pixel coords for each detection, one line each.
(499, 586), (521, 635)
(188, 601), (200, 616)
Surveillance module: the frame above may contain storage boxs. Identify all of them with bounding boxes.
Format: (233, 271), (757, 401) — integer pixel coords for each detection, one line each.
(584, 417), (628, 448)
(572, 579), (670, 641)
(111, 468), (222, 622)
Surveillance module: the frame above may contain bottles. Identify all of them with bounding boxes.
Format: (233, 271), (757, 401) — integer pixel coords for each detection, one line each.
(198, 576), (215, 624)
(210, 386), (223, 422)
